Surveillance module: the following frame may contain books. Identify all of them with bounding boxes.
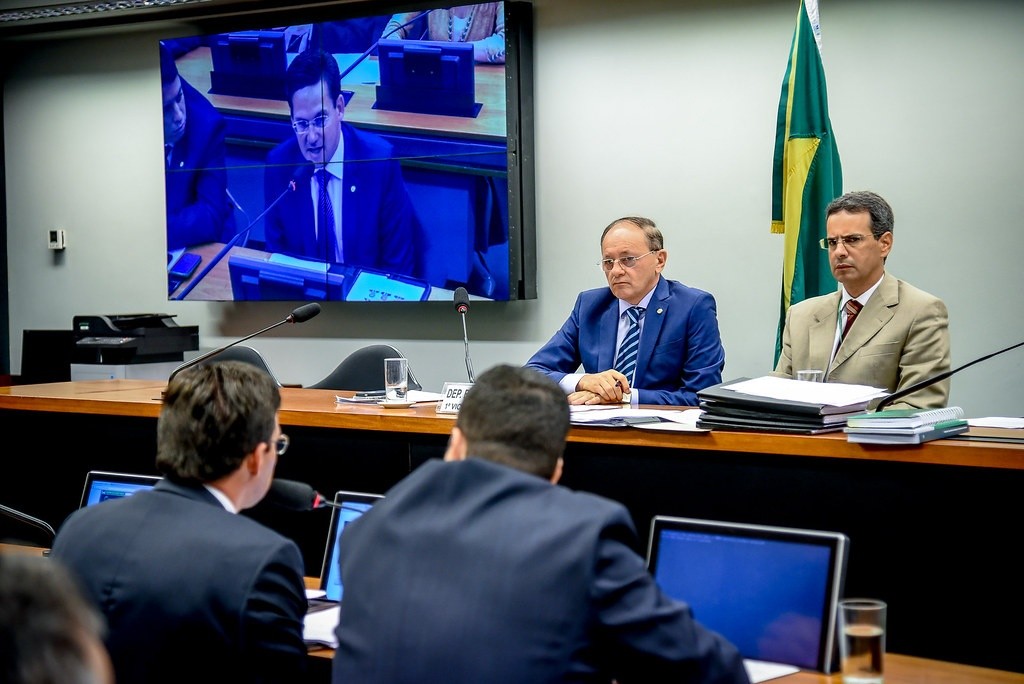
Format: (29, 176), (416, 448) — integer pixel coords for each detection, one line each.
(843, 406), (970, 445)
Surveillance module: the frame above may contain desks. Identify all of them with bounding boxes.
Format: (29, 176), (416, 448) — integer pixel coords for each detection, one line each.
(167, 230), (495, 301)
(0, 389), (1023, 675)
(177, 39), (507, 242)
(0, 543), (1024, 683)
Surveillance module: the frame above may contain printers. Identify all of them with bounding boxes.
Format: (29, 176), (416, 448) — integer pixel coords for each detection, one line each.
(72, 311), (199, 381)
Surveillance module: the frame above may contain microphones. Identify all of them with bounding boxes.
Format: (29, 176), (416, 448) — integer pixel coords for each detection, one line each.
(452, 286), (476, 384)
(175, 160), (315, 298)
(312, 494), (363, 516)
(164, 303), (321, 392)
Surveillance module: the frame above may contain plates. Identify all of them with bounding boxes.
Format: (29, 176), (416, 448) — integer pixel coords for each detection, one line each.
(377, 401), (416, 410)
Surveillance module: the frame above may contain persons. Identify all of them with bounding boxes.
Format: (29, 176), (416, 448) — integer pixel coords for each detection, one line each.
(48, 359), (310, 684)
(381, 0), (506, 65)
(767, 191), (949, 411)
(334, 365), (753, 684)
(0, 545), (112, 683)
(160, 42), (228, 251)
(522, 217), (726, 406)
(264, 47), (414, 278)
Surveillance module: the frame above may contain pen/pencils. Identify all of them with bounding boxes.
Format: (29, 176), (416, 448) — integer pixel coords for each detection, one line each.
(356, 393), (386, 397)
(615, 381), (621, 387)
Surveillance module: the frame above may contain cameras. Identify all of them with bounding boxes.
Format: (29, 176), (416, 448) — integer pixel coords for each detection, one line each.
(78, 471), (170, 509)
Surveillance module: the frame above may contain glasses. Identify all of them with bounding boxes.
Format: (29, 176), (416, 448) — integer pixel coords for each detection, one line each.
(291, 105), (339, 135)
(819, 232), (883, 250)
(602, 249), (658, 271)
(247, 434), (289, 455)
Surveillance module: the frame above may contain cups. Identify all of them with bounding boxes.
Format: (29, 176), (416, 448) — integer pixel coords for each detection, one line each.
(836, 600), (886, 684)
(798, 369), (823, 383)
(384, 358), (408, 402)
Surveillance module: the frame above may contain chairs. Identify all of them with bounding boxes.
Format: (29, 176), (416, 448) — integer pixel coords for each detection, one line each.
(200, 346), (287, 389)
(308, 343), (424, 393)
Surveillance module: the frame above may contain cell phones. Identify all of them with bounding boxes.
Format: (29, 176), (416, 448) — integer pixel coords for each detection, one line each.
(172, 253), (201, 279)
(355, 392), (386, 397)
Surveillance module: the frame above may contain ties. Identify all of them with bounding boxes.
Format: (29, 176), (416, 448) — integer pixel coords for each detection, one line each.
(164, 144), (173, 169)
(613, 306), (645, 389)
(313, 169), (340, 263)
(835, 300), (863, 358)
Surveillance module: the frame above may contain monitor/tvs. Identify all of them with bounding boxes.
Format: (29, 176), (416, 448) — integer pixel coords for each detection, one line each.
(158, 0), (521, 305)
(645, 518), (850, 676)
(208, 31), (291, 101)
(228, 255), (346, 303)
(376, 38), (476, 116)
(318, 491), (399, 602)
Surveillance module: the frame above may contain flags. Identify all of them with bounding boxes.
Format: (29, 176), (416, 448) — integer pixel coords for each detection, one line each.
(771, 0), (843, 373)
